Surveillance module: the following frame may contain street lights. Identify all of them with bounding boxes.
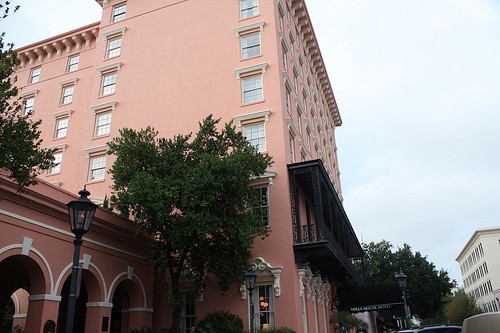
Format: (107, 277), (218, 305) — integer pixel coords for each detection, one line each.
(243, 267), (258, 332)
(63, 183), (100, 333)
(395, 265), (410, 329)
